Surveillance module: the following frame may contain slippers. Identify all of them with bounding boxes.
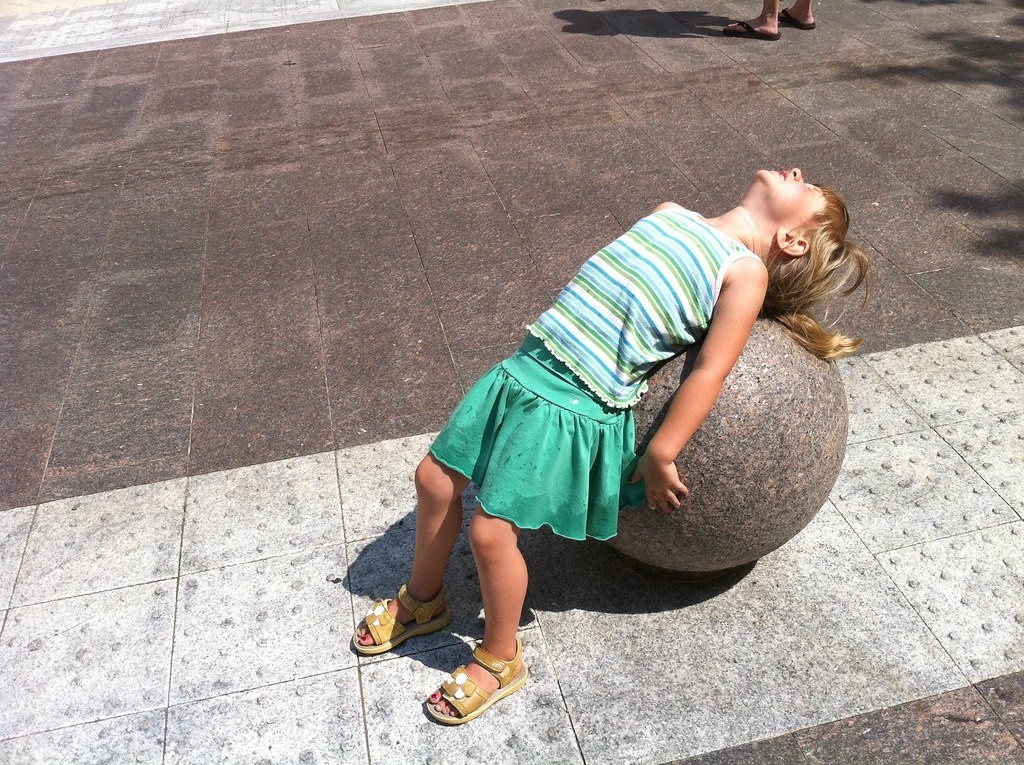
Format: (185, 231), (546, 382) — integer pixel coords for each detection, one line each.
(723, 21), (781, 40)
(778, 8), (816, 29)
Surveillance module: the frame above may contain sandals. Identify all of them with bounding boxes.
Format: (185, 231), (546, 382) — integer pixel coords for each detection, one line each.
(352, 583), (449, 654)
(426, 634), (528, 723)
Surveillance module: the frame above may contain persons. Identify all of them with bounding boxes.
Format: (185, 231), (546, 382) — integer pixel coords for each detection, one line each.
(723, 1), (816, 40)
(352, 167), (868, 726)
